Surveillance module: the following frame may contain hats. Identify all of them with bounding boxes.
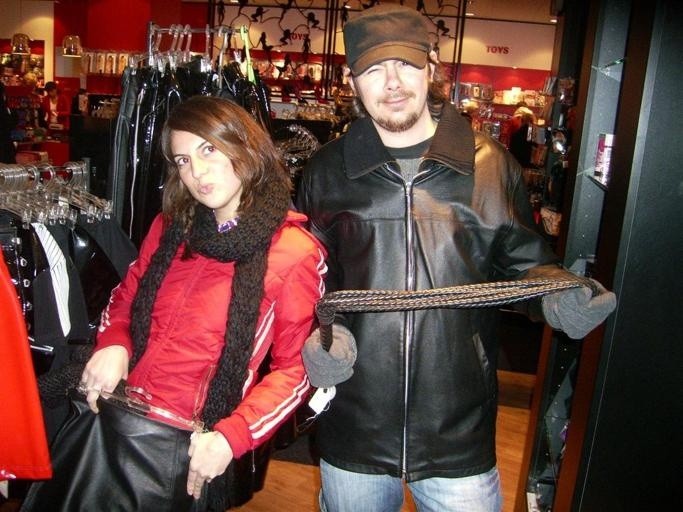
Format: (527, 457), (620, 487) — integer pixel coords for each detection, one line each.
(341, 5), (431, 77)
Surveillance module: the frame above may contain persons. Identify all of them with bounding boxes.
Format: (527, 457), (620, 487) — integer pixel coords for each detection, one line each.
(38, 82), (70, 127)
(280, 3), (617, 511)
(77, 94), (322, 508)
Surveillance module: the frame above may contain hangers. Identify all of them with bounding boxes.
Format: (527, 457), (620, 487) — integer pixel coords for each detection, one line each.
(0, 161), (117, 225)
(124, 18), (260, 91)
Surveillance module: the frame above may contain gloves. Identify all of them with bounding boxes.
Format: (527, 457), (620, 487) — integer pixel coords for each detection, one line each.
(540, 277), (618, 341)
(301, 321), (357, 388)
